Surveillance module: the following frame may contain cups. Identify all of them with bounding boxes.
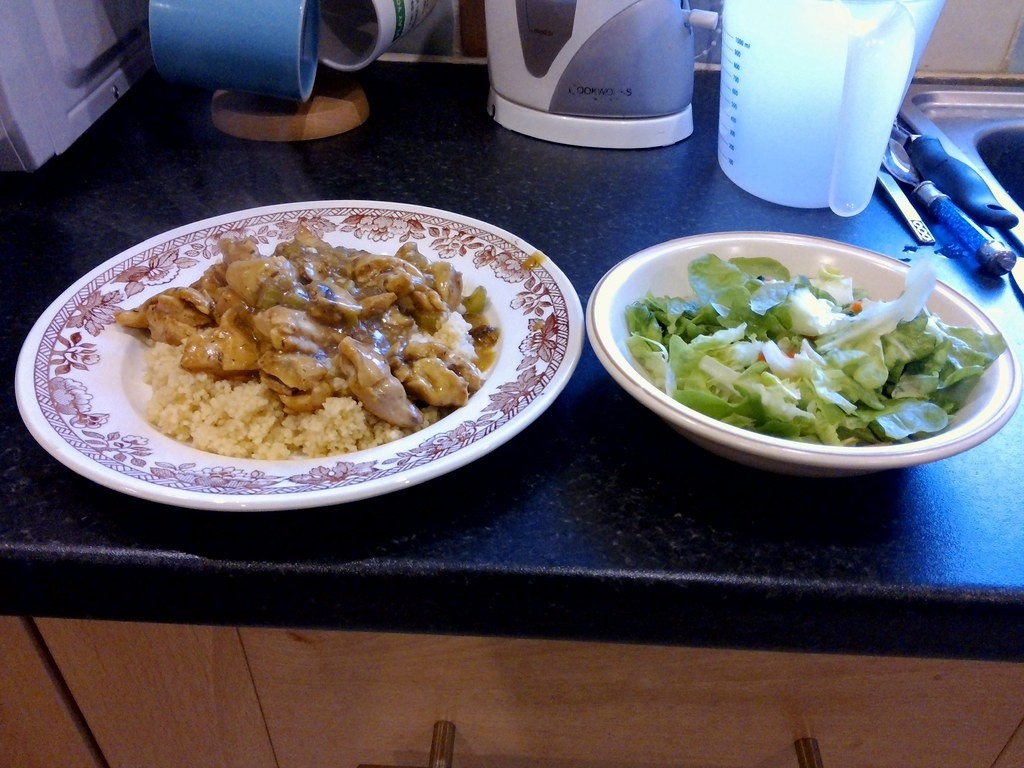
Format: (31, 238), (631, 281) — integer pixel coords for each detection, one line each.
(147, 0), (321, 104)
(319, 0), (439, 72)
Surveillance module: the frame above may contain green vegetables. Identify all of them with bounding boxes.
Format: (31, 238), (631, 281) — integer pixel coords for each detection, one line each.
(627, 252), (1007, 445)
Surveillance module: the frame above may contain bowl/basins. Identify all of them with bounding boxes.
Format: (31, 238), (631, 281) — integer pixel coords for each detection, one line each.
(585, 230), (1023, 478)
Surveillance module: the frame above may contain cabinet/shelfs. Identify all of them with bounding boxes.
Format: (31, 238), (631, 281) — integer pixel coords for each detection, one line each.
(0, 616), (1024, 768)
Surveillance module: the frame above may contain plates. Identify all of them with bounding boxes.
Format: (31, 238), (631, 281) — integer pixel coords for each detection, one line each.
(13, 197), (588, 515)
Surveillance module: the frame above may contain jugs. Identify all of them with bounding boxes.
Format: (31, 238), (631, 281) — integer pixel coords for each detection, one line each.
(715, 0), (949, 219)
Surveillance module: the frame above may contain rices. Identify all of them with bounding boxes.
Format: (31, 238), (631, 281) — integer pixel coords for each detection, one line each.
(141, 305), (477, 461)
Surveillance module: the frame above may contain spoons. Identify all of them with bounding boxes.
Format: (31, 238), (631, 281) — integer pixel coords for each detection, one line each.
(881, 137), (1018, 276)
